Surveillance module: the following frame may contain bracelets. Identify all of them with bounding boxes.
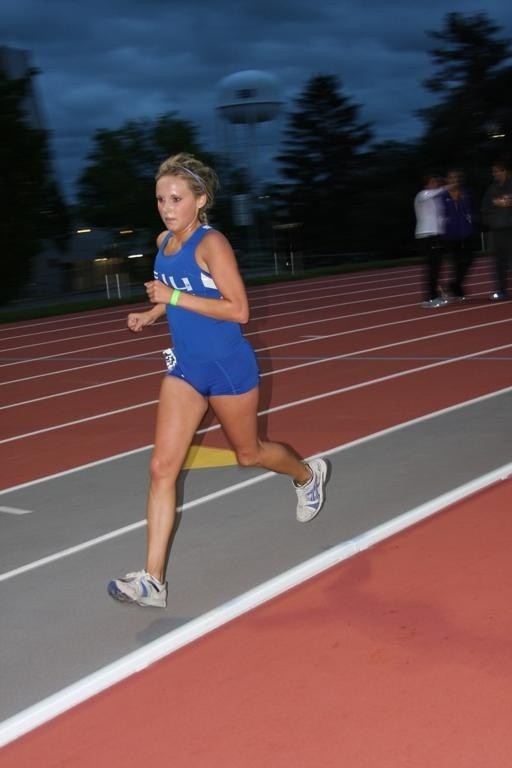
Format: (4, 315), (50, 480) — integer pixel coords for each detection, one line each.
(170, 288), (182, 306)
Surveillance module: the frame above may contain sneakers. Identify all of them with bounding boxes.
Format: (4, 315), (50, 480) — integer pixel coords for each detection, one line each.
(292, 456), (328, 524)
(423, 284), (467, 309)
(490, 290), (510, 300)
(107, 572), (168, 609)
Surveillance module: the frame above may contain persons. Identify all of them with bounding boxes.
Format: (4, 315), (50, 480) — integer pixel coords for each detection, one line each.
(439, 168), (481, 304)
(104, 153), (330, 610)
(412, 173), (459, 310)
(479, 161), (512, 303)
